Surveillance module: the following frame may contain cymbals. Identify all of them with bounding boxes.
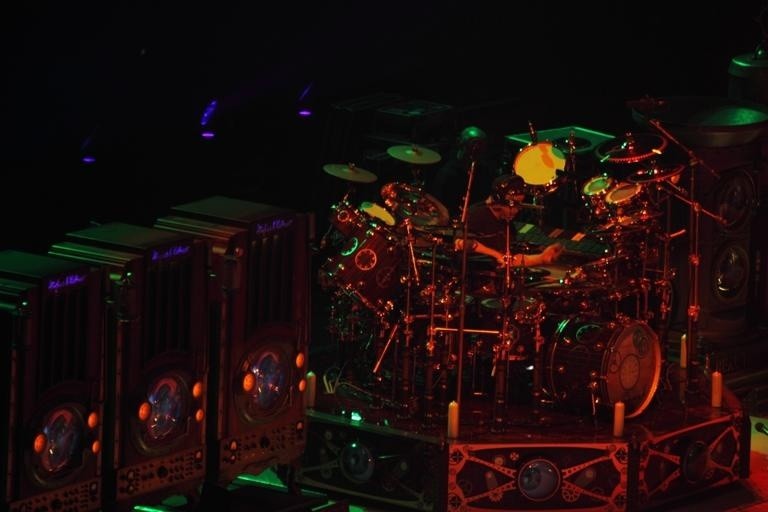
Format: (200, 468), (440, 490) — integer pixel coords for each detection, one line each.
(380, 182), (450, 227)
(596, 133), (685, 183)
(386, 144), (443, 164)
(324, 164), (377, 182)
(399, 236), (435, 248)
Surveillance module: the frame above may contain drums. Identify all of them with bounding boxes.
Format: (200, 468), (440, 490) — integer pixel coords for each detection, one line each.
(320, 220), (415, 317)
(512, 139), (569, 199)
(407, 253), (641, 362)
(548, 313), (662, 418)
(329, 200), (368, 237)
(580, 176), (664, 228)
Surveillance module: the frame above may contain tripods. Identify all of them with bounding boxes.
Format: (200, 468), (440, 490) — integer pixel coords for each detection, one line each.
(463, 216), (551, 441)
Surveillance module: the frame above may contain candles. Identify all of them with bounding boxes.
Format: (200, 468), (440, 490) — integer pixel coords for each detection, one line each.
(305, 370), (316, 408)
(613, 401), (625, 438)
(447, 400), (459, 439)
(680, 336), (687, 369)
(712, 371), (724, 407)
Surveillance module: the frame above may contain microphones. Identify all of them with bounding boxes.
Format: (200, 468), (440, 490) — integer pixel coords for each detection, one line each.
(568, 131), (577, 155)
(527, 119), (537, 142)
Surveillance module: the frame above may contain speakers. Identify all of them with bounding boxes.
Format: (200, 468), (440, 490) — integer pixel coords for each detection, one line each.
(152, 196), (313, 485)
(0, 249), (109, 512)
(604, 136), (761, 337)
(47, 219), (210, 512)
